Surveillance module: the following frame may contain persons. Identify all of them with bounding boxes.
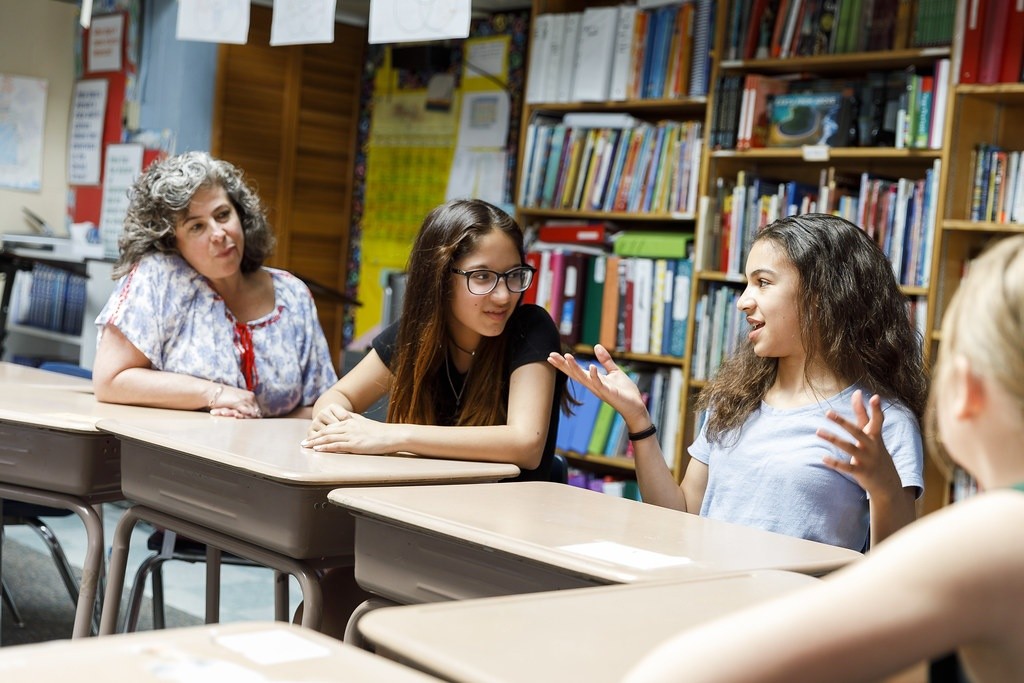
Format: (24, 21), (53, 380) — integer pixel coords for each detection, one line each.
(295, 196), (584, 644)
(92, 150), (341, 421)
(625, 237), (1024, 683)
(547, 214), (927, 554)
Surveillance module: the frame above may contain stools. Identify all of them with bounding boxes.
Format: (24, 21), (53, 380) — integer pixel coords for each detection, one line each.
(122, 532), (289, 633)
(0, 497), (106, 636)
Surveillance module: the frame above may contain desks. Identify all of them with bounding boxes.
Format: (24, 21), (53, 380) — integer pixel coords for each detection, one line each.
(0, 363), (928, 683)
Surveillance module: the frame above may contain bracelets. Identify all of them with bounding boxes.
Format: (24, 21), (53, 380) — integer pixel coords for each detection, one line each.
(629, 424), (657, 441)
(208, 387), (223, 413)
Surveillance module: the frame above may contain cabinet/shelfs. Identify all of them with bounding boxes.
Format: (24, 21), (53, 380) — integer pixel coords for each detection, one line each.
(0, 235), (125, 371)
(514, 0), (1024, 555)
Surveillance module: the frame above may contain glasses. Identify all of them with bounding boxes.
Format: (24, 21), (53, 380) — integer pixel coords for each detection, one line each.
(446, 263), (538, 296)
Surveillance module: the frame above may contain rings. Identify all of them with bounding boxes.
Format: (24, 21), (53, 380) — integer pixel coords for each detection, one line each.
(255, 409), (261, 413)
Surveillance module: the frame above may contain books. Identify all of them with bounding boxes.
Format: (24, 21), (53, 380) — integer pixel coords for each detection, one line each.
(5, 262), (87, 337)
(517, 0), (1024, 503)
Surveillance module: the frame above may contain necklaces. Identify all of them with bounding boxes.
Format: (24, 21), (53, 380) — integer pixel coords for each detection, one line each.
(445, 339), (475, 406)
